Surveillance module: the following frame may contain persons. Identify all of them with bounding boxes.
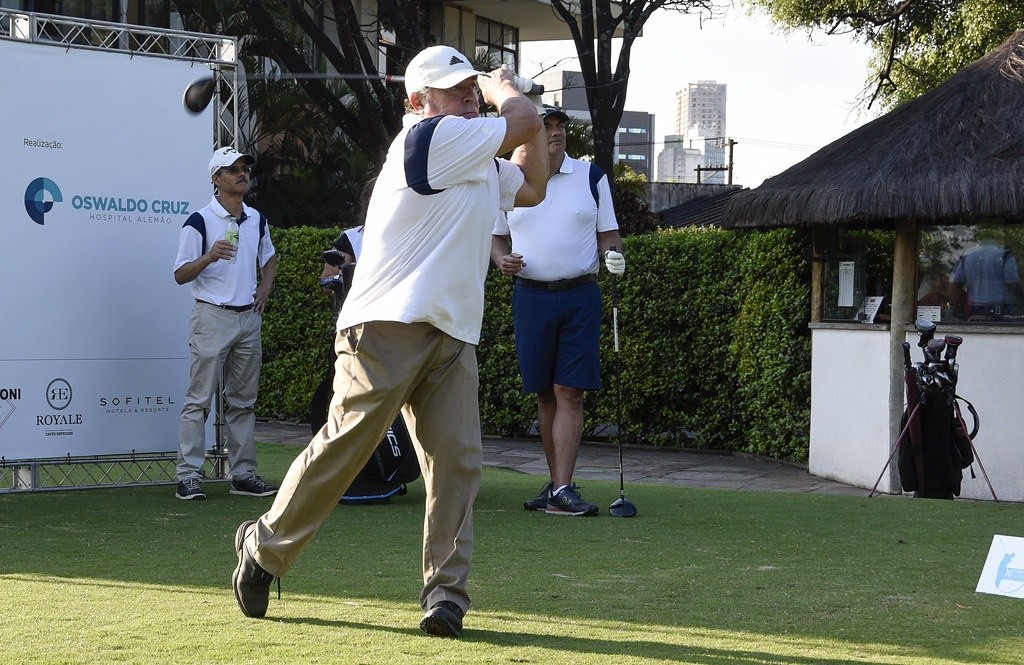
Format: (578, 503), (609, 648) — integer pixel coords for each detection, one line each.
(492, 105), (626, 514)
(172, 147), (279, 500)
(232, 45), (549, 655)
(319, 176), (378, 279)
(917, 242), (1024, 323)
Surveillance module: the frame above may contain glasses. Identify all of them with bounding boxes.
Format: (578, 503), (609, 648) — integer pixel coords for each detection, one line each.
(218, 167), (252, 176)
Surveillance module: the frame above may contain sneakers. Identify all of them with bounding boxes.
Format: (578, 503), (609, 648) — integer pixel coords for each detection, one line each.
(524, 481), (553, 510)
(545, 483), (598, 515)
(232, 520), (280, 618)
(229, 474), (279, 497)
(176, 478), (207, 500)
(419, 601), (464, 636)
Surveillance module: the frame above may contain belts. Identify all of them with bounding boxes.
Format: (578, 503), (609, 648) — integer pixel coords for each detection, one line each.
(515, 274), (596, 290)
(197, 299), (255, 312)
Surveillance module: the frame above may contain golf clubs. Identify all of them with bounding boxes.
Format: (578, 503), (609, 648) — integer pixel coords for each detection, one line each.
(914, 317), (964, 387)
(608, 243), (640, 520)
(182, 71), (546, 115)
(320, 249), (357, 299)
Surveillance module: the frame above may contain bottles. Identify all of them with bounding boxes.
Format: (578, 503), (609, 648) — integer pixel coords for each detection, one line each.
(225, 217), (239, 264)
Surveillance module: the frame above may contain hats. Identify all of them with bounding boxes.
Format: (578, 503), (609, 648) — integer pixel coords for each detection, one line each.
(405, 45), (491, 101)
(209, 146), (256, 183)
(543, 104), (570, 121)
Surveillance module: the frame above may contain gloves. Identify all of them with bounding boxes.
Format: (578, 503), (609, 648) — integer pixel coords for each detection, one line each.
(605, 250), (626, 276)
(512, 75), (547, 119)
